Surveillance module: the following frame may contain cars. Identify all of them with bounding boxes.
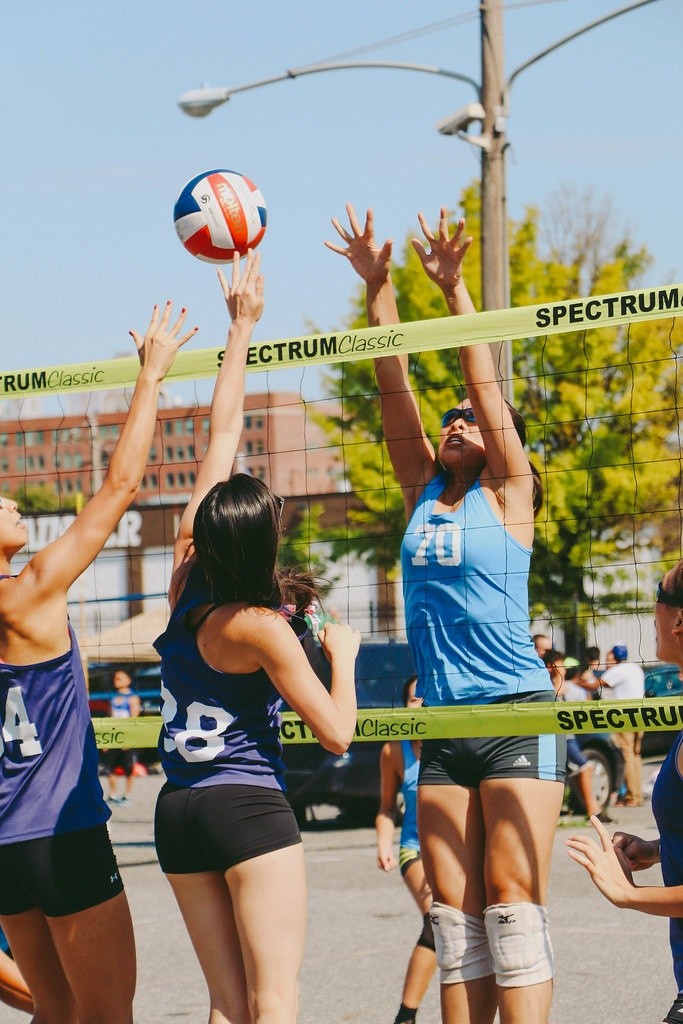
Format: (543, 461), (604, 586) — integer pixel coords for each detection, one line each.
(595, 664), (683, 760)
(92, 661), (165, 769)
(274, 637), (627, 827)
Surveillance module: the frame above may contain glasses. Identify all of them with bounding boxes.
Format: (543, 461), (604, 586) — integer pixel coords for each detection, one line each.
(654, 580), (682, 608)
(273, 494), (285, 518)
(441, 407), (476, 427)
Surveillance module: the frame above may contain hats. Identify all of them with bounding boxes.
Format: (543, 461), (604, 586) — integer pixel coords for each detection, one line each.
(611, 646), (626, 659)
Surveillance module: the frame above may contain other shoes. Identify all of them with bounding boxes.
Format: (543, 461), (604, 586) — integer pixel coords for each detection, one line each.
(116, 796), (130, 806)
(587, 811), (614, 826)
(615, 796), (643, 807)
(104, 797), (116, 805)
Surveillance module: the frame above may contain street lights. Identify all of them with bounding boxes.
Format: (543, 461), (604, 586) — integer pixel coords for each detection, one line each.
(177, 59), (514, 409)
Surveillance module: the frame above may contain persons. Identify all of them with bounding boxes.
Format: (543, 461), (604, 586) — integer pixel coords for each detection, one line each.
(563, 565), (683, 1024)
(103, 669), (141, 807)
(531, 634), (644, 825)
(324, 202), (567, 1024)
(0, 300), (199, 1024)
(376, 675), (438, 1024)
(149, 248), (362, 1024)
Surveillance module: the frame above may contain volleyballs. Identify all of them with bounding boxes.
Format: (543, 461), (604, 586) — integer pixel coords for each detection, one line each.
(173, 168), (268, 266)
